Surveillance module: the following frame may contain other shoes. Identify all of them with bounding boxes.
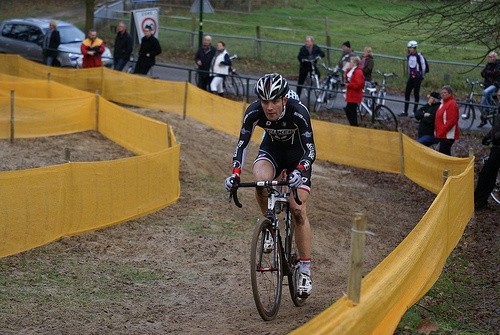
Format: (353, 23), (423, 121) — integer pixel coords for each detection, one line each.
(476, 121), (488, 128)
(211, 91), (224, 97)
(397, 112), (408, 117)
(409, 113), (415, 118)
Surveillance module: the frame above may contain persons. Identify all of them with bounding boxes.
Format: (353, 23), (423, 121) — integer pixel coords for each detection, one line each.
(434, 85), (459, 156)
(114, 22), (134, 71)
(42, 21), (60, 66)
(81, 30), (105, 68)
(415, 92), (442, 147)
(209, 41), (232, 97)
(361, 46), (374, 82)
(134, 26), (161, 75)
(194, 35), (216, 94)
(225, 73), (316, 298)
(474, 104), (500, 211)
(397, 40), (426, 118)
(296, 35), (327, 103)
(339, 41), (354, 81)
(477, 51), (500, 128)
(344, 55), (364, 126)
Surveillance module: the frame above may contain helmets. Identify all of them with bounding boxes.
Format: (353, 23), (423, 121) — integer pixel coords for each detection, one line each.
(254, 73), (289, 101)
(407, 40), (418, 48)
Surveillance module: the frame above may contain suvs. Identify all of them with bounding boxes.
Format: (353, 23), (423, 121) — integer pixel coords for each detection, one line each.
(0, 17), (113, 68)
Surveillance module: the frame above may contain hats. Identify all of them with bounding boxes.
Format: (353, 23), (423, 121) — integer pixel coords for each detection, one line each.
(430, 91), (441, 100)
(344, 40), (350, 48)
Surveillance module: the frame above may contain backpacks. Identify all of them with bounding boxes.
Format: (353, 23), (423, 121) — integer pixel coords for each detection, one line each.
(416, 52), (429, 73)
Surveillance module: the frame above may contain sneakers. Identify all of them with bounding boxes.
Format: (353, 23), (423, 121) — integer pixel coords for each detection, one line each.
(297, 269), (313, 297)
(263, 228), (274, 254)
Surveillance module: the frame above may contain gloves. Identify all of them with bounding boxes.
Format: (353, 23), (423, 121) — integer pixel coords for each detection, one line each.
(288, 170), (303, 188)
(224, 173), (240, 192)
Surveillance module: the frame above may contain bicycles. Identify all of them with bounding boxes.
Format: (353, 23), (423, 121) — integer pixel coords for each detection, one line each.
(358, 70), (398, 131)
(229, 173), (307, 321)
(480, 136), (500, 205)
(303, 57), (340, 112)
(456, 79), (496, 132)
(194, 54), (245, 96)
